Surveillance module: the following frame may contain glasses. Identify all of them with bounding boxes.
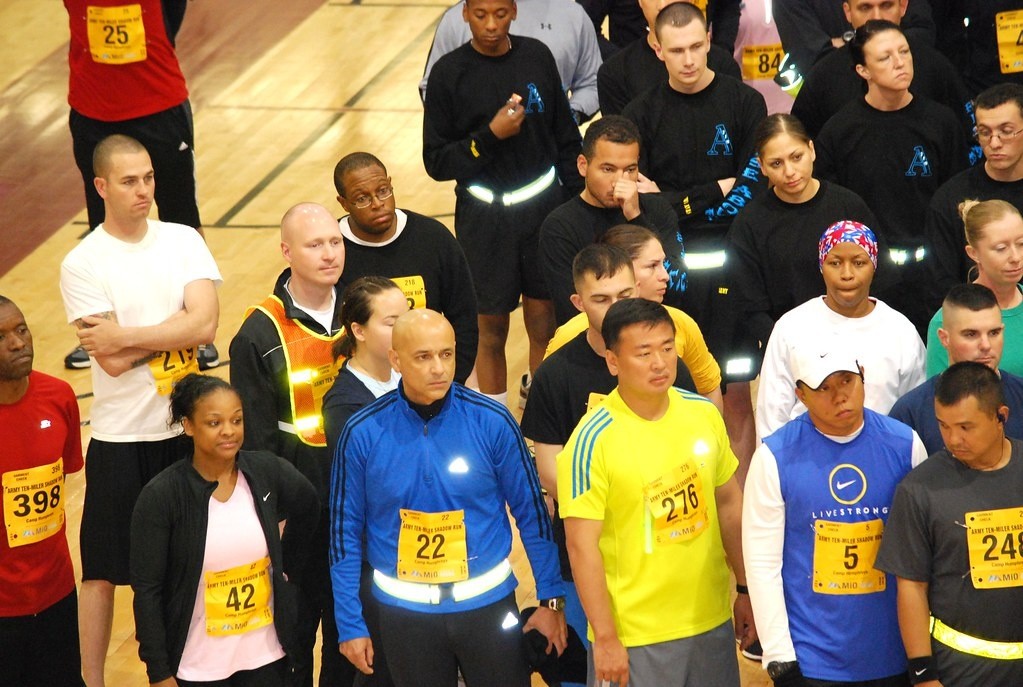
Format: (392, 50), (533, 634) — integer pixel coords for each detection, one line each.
(346, 182), (393, 208)
(972, 128), (1022, 145)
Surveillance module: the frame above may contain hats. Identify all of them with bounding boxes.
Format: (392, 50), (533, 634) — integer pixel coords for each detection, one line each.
(789, 335), (861, 390)
(818, 220), (878, 272)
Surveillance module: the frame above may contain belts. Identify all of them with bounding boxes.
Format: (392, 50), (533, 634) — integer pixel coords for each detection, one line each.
(465, 164), (557, 206)
(366, 559), (513, 606)
(887, 245), (931, 264)
(684, 249), (727, 270)
(928, 615), (1023, 660)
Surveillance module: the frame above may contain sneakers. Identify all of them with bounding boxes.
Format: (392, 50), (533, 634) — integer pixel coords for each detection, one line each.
(195, 343), (220, 369)
(64, 343), (91, 369)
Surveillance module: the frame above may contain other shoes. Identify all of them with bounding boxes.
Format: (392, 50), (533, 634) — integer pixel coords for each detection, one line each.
(737, 632), (763, 663)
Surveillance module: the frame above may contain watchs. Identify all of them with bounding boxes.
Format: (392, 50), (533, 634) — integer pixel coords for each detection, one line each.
(767, 661), (799, 679)
(539, 596), (566, 612)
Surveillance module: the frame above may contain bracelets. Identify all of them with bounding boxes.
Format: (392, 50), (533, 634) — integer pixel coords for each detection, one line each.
(736, 585), (748, 594)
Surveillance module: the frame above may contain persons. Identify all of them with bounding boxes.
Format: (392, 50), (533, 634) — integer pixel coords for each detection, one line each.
(742, 334), (1023, 687)
(424, 0), (1023, 411)
(925, 83), (1023, 380)
(231, 204), (381, 687)
(58, 0), (220, 370)
(335, 152), (480, 386)
(0, 295), (85, 687)
(130, 293), (758, 687)
(420, 0), (1023, 127)
